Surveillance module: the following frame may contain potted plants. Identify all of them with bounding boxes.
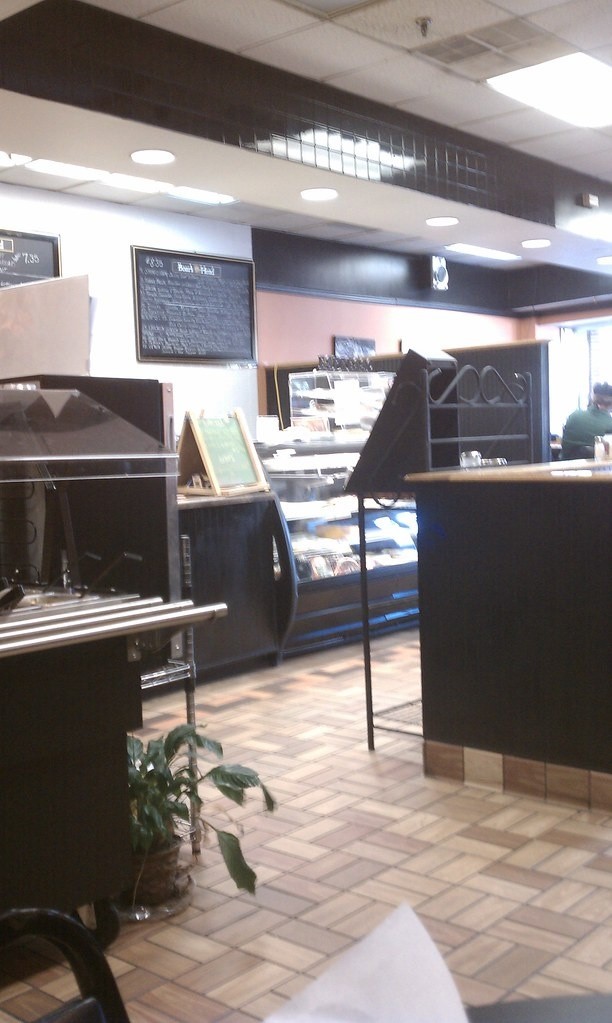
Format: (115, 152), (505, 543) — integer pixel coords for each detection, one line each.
(121, 722), (274, 906)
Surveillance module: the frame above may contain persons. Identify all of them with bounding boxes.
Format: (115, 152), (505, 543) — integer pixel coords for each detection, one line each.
(559, 382), (612, 461)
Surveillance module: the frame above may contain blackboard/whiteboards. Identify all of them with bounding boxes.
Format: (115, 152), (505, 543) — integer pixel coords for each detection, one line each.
(130, 243), (258, 365)
(0, 230), (63, 289)
(186, 409), (267, 495)
